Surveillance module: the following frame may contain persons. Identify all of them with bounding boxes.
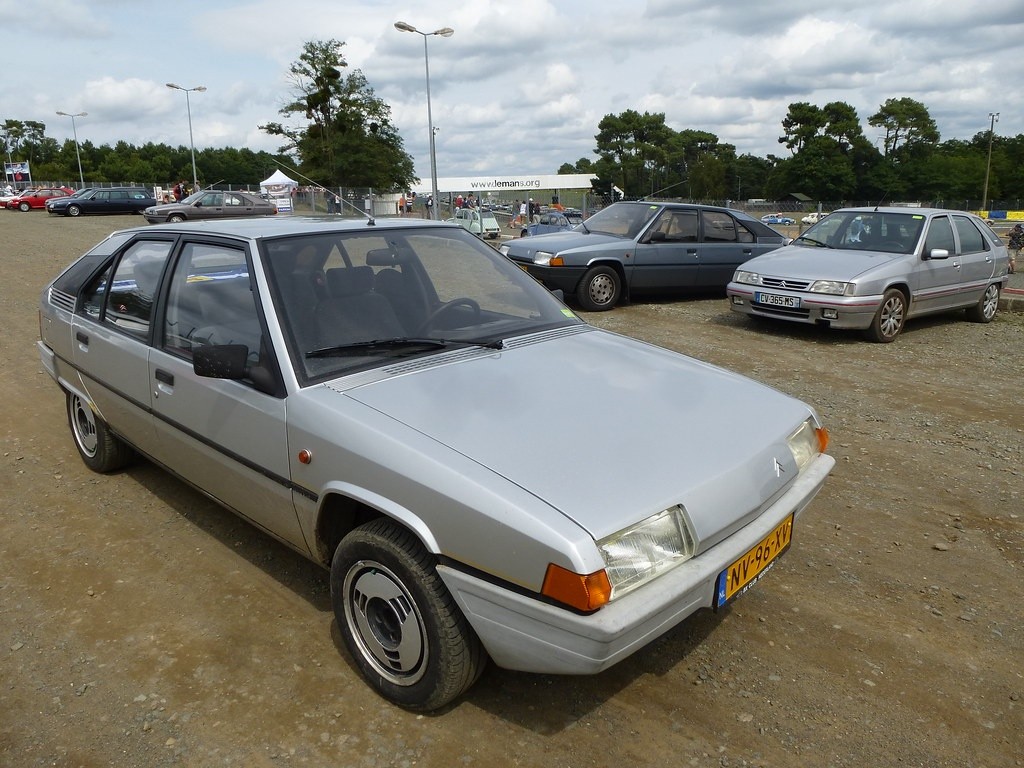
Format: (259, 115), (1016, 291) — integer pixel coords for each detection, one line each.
(512, 199), (540, 223)
(162, 195), (169, 204)
(399, 192), (416, 213)
(20, 186), (24, 192)
(173, 180), (201, 201)
(456, 194), (479, 210)
(291, 186), (304, 209)
(327, 192), (342, 215)
(1007, 224), (1024, 274)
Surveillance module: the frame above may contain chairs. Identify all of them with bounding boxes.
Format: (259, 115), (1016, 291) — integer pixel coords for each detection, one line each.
(191, 285), (262, 362)
(317, 265), (410, 345)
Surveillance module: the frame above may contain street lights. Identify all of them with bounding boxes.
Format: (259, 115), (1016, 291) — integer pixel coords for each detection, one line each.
(166, 81), (208, 195)
(982, 112), (1001, 211)
(56, 110), (88, 189)
(394, 21), (455, 220)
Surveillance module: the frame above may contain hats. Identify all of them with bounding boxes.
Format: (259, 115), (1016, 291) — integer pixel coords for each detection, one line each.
(1015, 224), (1024, 229)
(457, 195), (462, 199)
(529, 198), (533, 201)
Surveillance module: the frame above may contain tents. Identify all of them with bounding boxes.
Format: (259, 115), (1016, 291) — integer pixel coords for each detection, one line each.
(260, 169), (298, 198)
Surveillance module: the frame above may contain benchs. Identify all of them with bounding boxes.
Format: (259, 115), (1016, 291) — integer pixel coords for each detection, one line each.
(134, 247), (315, 333)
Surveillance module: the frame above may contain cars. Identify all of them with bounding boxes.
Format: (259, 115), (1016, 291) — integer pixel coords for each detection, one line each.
(519, 205), (601, 238)
(981, 216), (995, 227)
(417, 193), (513, 239)
(727, 205), (1011, 343)
(143, 189), (278, 225)
(0, 185), (178, 217)
(761, 213), (795, 227)
(36, 215), (838, 715)
(802, 212), (829, 225)
(500, 200), (804, 312)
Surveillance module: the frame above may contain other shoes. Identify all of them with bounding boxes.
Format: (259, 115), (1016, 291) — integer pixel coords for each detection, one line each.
(1008, 266), (1010, 274)
(1011, 269), (1016, 274)
